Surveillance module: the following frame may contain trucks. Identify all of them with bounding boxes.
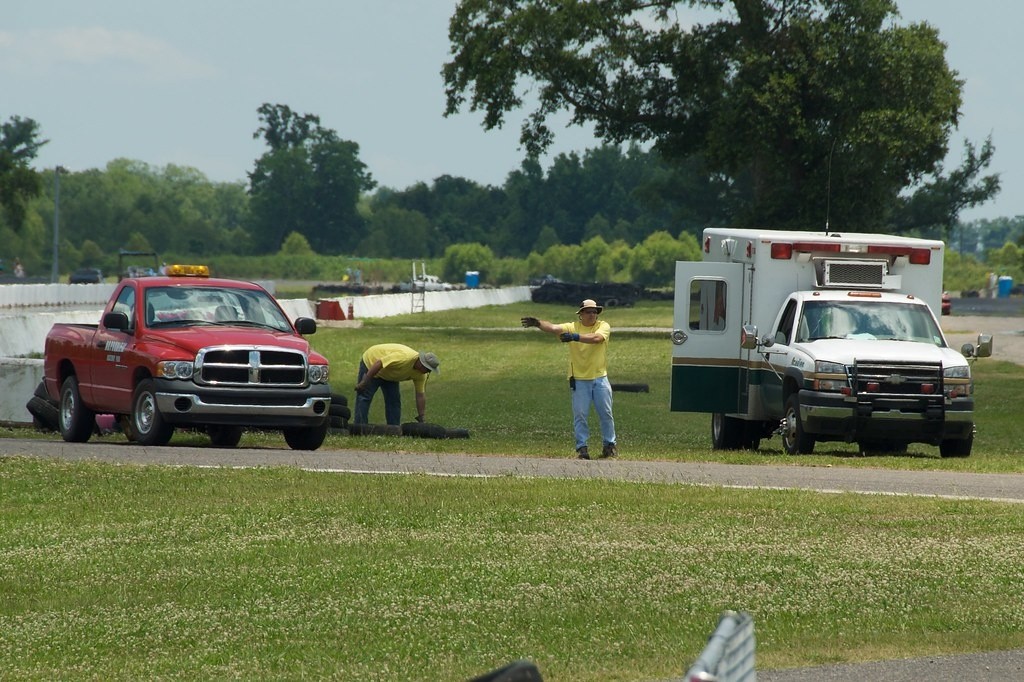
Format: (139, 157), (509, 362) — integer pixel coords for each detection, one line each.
(669, 226), (994, 456)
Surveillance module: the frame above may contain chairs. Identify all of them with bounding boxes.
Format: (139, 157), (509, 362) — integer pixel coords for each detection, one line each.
(131, 302), (155, 324)
(216, 305), (239, 321)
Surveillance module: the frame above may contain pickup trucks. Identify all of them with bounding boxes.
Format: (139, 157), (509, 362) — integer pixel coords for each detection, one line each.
(399, 275), (452, 293)
(43, 264), (333, 447)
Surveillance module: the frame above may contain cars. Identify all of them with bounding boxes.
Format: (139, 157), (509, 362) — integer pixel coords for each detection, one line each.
(119, 264), (157, 284)
(69, 267), (106, 284)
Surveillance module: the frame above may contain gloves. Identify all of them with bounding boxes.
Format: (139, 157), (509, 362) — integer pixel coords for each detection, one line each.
(520, 317), (541, 328)
(560, 332), (580, 343)
(415, 413), (426, 422)
(353, 373), (371, 393)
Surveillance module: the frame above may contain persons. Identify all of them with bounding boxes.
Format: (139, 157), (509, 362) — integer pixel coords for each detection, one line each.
(353, 343), (439, 426)
(13, 256), (25, 279)
(157, 261), (167, 276)
(0, 258), (8, 277)
(144, 265), (154, 276)
(521, 299), (618, 460)
(128, 265), (139, 278)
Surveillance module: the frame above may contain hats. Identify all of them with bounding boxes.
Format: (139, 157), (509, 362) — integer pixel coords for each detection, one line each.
(418, 351), (441, 375)
(576, 299), (603, 316)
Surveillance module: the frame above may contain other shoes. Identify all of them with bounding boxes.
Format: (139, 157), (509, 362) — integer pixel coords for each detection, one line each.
(578, 447), (589, 459)
(603, 445), (616, 458)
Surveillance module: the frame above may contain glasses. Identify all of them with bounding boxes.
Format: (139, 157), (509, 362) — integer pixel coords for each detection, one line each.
(583, 310), (599, 315)
